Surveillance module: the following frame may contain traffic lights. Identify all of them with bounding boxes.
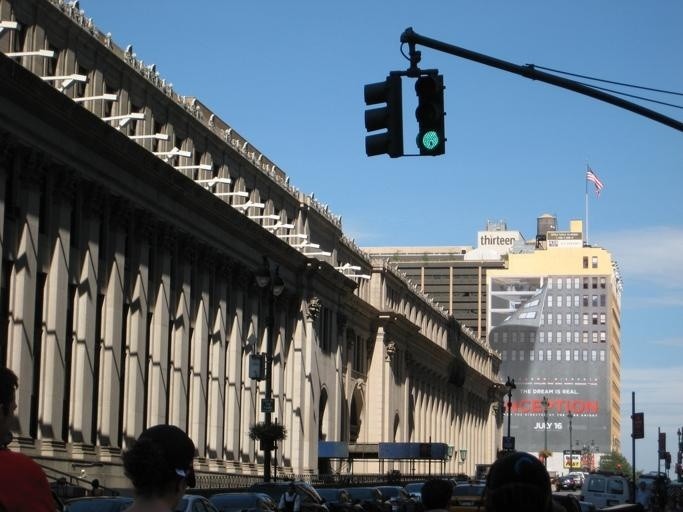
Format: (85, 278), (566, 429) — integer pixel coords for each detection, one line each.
(361, 73), (406, 159)
(414, 71), (448, 158)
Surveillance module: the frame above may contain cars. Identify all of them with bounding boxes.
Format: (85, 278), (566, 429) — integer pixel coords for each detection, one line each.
(550, 469), (665, 509)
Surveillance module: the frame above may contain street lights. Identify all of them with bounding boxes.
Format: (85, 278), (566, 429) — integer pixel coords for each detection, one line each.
(541, 395), (549, 468)
(255, 250), (285, 480)
(567, 410), (573, 470)
(504, 375), (516, 452)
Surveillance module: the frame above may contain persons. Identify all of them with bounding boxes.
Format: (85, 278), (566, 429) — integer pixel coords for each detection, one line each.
(121, 424), (196, 512)
(636, 482), (654, 507)
(480, 451), (553, 512)
(339, 479), (452, 512)
(0, 367), (59, 512)
(277, 483), (301, 512)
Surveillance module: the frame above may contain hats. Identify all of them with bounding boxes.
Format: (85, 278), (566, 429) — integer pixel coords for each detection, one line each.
(132, 423), (197, 490)
(487, 450), (552, 493)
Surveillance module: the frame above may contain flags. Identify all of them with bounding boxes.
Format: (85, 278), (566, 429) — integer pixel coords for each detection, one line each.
(586, 167), (604, 199)
